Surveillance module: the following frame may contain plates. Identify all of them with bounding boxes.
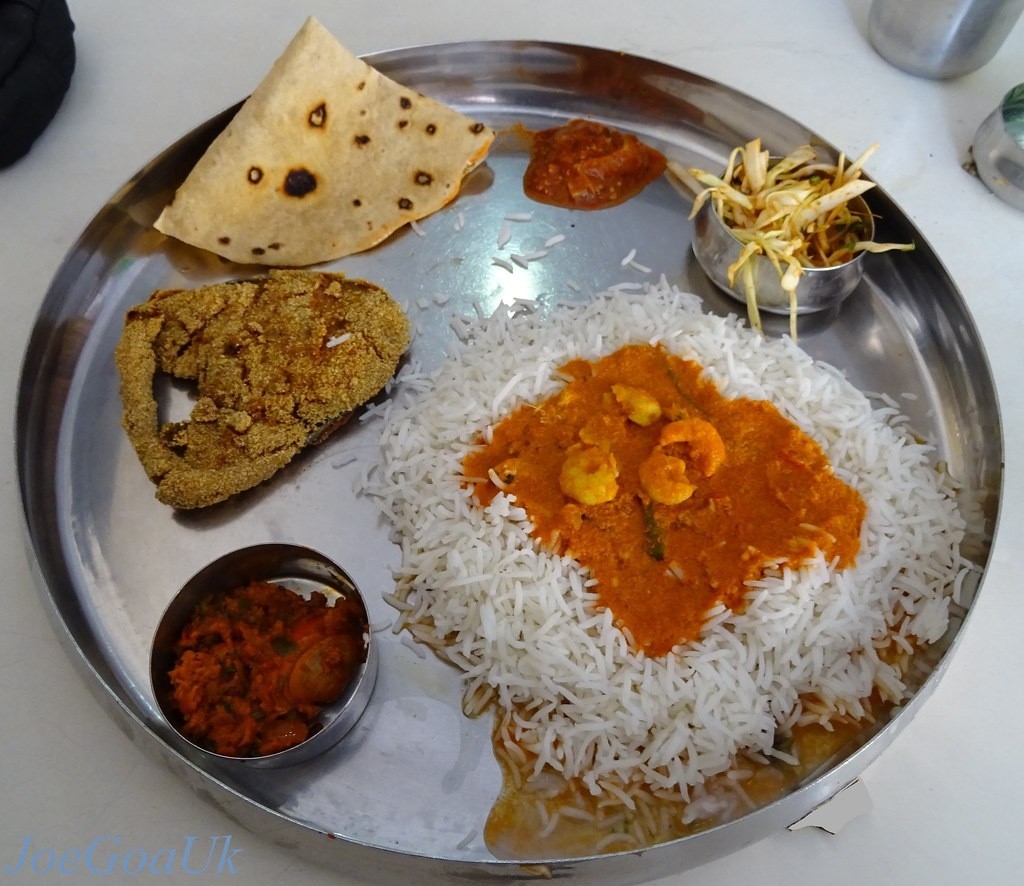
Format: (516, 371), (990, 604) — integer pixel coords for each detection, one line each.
(13, 38), (1008, 886)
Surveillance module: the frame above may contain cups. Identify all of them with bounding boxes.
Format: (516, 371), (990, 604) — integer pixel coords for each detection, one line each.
(868, 1), (1023, 82)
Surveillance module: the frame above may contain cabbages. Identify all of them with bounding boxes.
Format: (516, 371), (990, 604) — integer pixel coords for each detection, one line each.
(695, 144), (912, 341)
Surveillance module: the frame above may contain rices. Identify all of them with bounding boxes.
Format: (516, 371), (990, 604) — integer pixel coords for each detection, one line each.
(331, 208), (990, 851)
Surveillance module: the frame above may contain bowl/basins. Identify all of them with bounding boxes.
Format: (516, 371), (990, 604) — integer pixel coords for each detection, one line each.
(689, 156), (875, 316)
(148, 542), (379, 772)
(972, 83), (1024, 214)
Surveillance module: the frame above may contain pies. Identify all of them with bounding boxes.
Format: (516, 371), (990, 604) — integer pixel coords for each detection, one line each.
(154, 17), (494, 266)
